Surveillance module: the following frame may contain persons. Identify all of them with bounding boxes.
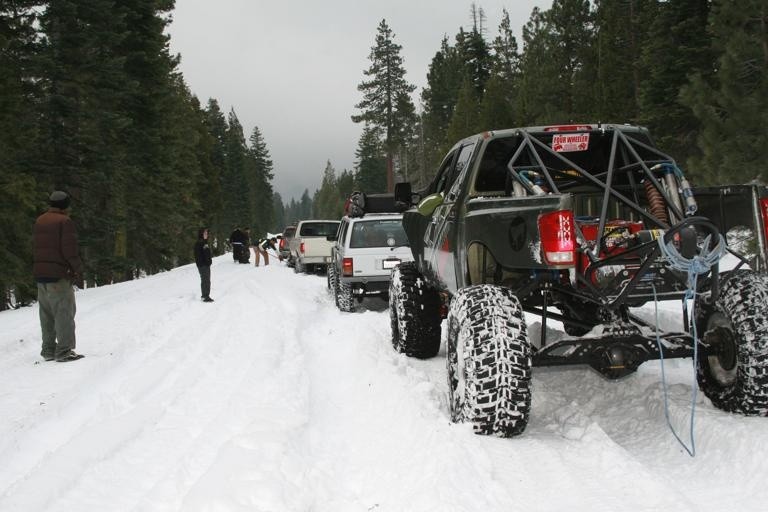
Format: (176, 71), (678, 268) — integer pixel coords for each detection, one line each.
(33, 191), (85, 364)
(253, 237), (283, 267)
(229, 224), (246, 264)
(193, 226), (216, 302)
(241, 227), (251, 263)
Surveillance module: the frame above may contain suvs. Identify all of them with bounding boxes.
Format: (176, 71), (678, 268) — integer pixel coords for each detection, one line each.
(277, 212), (418, 314)
(391, 122), (767, 440)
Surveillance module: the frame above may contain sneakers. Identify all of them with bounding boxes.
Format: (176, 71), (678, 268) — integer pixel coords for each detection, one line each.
(201, 296), (213, 301)
(46, 351), (84, 362)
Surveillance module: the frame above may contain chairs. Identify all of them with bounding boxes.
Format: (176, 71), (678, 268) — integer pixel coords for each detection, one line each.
(353, 229), (408, 248)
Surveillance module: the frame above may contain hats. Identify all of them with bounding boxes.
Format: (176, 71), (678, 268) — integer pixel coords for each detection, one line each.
(47, 191), (71, 209)
